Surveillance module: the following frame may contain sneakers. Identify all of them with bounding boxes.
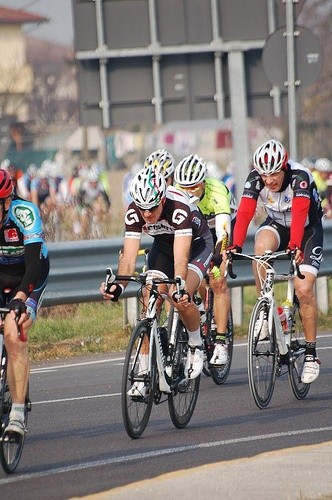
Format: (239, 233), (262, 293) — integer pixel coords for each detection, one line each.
(127, 373), (150, 397)
(184, 344), (203, 380)
(209, 344), (229, 366)
(251, 312), (268, 341)
(300, 355), (320, 384)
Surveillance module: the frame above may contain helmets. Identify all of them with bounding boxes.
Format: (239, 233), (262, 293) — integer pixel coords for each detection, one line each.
(289, 157), (332, 172)
(143, 149), (175, 179)
(28, 160), (100, 182)
(0, 168), (14, 198)
(173, 154), (207, 188)
(129, 168), (167, 209)
(253, 139), (288, 176)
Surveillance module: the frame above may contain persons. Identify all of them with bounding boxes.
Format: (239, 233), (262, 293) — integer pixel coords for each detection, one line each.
(173, 155), (238, 365)
(0, 170), (50, 441)
(102, 167), (214, 396)
(221, 140), (324, 384)
(0, 159), (332, 241)
(143, 149), (176, 188)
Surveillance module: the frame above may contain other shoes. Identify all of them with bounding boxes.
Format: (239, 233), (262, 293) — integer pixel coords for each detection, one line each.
(4, 419), (26, 436)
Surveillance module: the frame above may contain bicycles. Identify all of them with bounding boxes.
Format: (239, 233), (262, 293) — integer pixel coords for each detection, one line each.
(104, 266), (202, 441)
(226, 248), (311, 410)
(0, 306), (31, 474)
(135, 249), (235, 396)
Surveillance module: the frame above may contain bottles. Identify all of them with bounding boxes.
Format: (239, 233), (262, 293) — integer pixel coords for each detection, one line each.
(281, 299), (292, 334)
(277, 306), (288, 330)
(193, 294), (207, 323)
(157, 326), (169, 356)
